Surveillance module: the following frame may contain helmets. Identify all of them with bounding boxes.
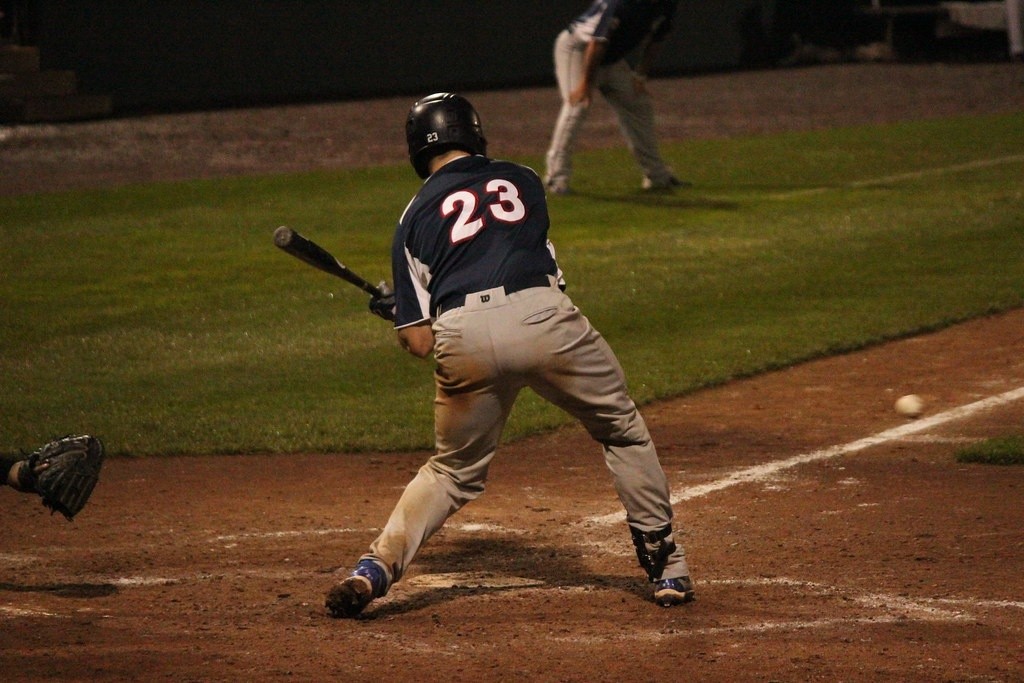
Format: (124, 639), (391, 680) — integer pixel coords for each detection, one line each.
(407, 94), (488, 178)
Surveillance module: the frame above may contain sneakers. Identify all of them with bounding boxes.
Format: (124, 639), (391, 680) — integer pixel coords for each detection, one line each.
(654, 576), (693, 607)
(545, 175), (570, 194)
(642, 177), (692, 190)
(325, 565), (385, 616)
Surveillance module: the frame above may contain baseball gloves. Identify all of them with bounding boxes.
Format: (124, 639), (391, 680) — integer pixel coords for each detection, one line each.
(29, 435), (106, 519)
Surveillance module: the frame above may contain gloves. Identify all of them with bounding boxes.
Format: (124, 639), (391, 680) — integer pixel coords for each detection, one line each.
(369, 282), (396, 322)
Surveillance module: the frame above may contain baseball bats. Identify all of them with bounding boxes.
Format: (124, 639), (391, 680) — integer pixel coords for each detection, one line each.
(272, 226), (381, 301)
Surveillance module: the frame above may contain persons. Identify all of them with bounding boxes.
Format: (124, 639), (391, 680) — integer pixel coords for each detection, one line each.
(324, 92), (693, 621)
(543, 0), (695, 197)
(0, 434), (103, 520)
